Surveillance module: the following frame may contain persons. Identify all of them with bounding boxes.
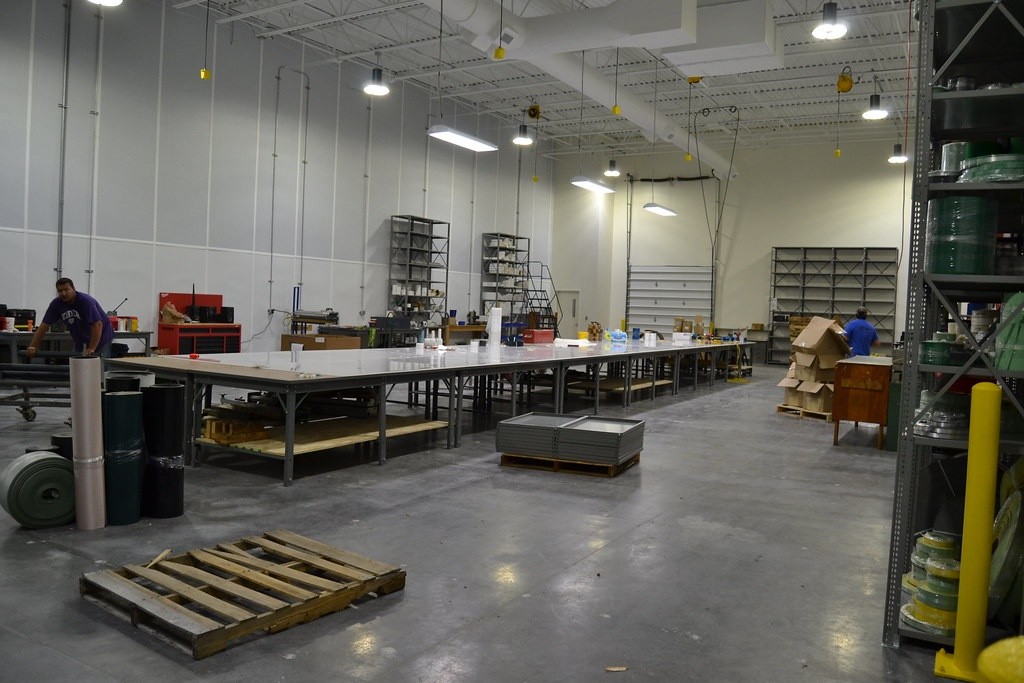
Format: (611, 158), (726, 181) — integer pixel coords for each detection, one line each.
(844, 307), (880, 357)
(26, 278), (114, 358)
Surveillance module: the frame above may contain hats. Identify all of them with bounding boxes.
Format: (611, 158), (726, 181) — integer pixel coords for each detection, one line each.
(857, 307), (867, 316)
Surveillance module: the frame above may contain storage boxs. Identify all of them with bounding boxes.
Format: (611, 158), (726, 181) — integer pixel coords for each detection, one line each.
(777, 315), (853, 411)
(523, 328), (554, 344)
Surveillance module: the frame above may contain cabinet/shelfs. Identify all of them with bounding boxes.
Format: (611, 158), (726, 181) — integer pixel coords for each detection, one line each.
(481, 232), (530, 347)
(878, 0), (1024, 650)
(832, 354), (893, 449)
(157, 292), (242, 355)
(764, 245), (898, 365)
(388, 214), (449, 345)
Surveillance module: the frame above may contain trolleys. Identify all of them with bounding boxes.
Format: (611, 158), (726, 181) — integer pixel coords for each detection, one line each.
(0, 349), (84, 429)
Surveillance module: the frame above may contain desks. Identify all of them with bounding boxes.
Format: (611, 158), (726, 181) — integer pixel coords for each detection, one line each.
(442, 323), (486, 346)
(281, 334), (360, 351)
(102, 341), (756, 488)
(0, 332), (152, 365)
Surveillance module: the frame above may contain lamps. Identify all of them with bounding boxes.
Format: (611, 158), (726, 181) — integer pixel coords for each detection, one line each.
(888, 136), (908, 164)
(604, 150), (620, 176)
(427, 1), (498, 152)
(572, 48), (616, 193)
(642, 60), (678, 216)
(812, 0), (847, 39)
(861, 80), (888, 119)
(364, 52), (391, 94)
(512, 110), (533, 144)
